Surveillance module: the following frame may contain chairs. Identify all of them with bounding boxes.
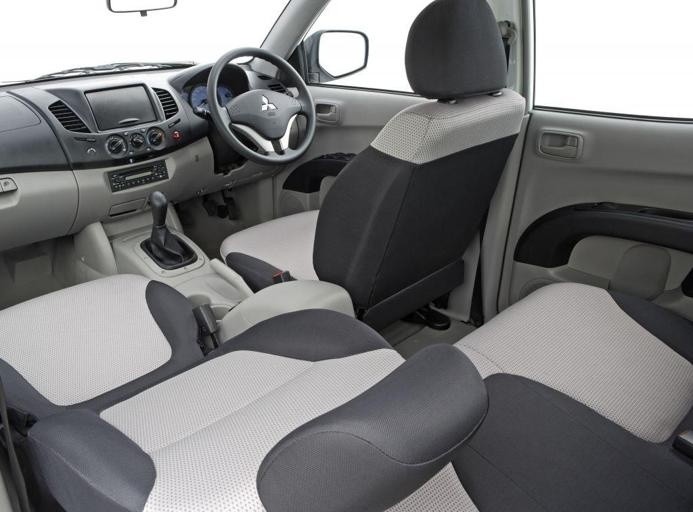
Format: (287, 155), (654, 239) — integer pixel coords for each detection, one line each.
(446, 280), (693, 510)
(215, 1), (527, 335)
(1, 271), (493, 511)
